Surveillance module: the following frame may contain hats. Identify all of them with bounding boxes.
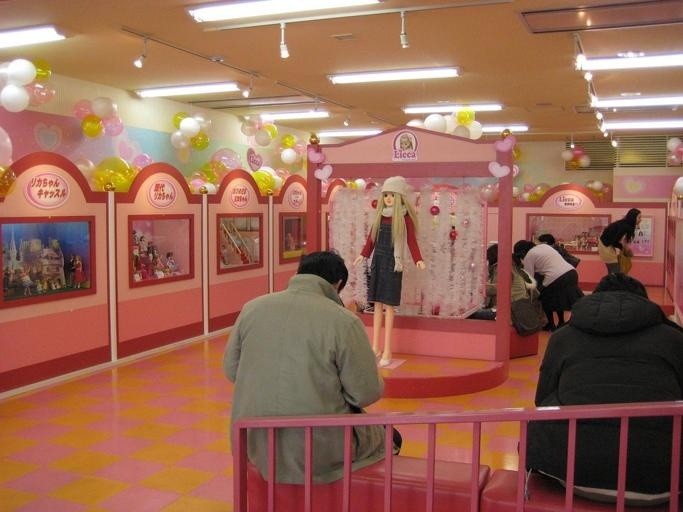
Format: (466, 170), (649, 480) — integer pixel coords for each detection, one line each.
(381, 175), (405, 194)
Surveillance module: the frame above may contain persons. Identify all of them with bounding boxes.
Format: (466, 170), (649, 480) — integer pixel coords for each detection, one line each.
(352, 176), (426, 367)
(222, 251), (402, 486)
(513, 240), (584, 332)
(468, 244), (545, 337)
(517, 272), (683, 511)
(4, 229), (177, 295)
(536, 233), (581, 269)
(598, 208), (641, 274)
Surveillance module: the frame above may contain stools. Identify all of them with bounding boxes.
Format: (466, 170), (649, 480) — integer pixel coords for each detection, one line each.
(509, 327), (539, 357)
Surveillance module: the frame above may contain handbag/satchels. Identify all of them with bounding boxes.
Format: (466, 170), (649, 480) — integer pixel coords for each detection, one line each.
(617, 252), (632, 274)
(510, 298), (541, 335)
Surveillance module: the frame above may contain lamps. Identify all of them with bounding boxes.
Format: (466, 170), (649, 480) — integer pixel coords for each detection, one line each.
(590, 93), (683, 110)
(1, 23), (73, 50)
(133, 36), (149, 68)
(186, 2), (511, 35)
(568, 71), (619, 150)
(343, 110), (352, 127)
(243, 77), (254, 100)
(134, 79), (241, 99)
(403, 100), (505, 115)
(325, 66), (464, 87)
(481, 124), (531, 135)
(398, 13), (411, 48)
(573, 49), (683, 72)
(314, 128), (383, 138)
(278, 24), (289, 60)
(239, 111), (332, 122)
(596, 119), (683, 132)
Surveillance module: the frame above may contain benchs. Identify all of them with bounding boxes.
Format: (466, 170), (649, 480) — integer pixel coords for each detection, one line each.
(479, 464), (676, 512)
(239, 449), (488, 512)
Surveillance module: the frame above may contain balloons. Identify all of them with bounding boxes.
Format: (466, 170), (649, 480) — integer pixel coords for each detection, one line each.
(73, 97), (124, 139)
(171, 110), (215, 161)
(354, 179), (366, 197)
(1, 57), (56, 113)
(273, 134), (304, 171)
(405, 107), (683, 202)
(76, 153), (153, 192)
(241, 117), (278, 146)
(188, 147), (290, 194)
(1, 127), (16, 197)
